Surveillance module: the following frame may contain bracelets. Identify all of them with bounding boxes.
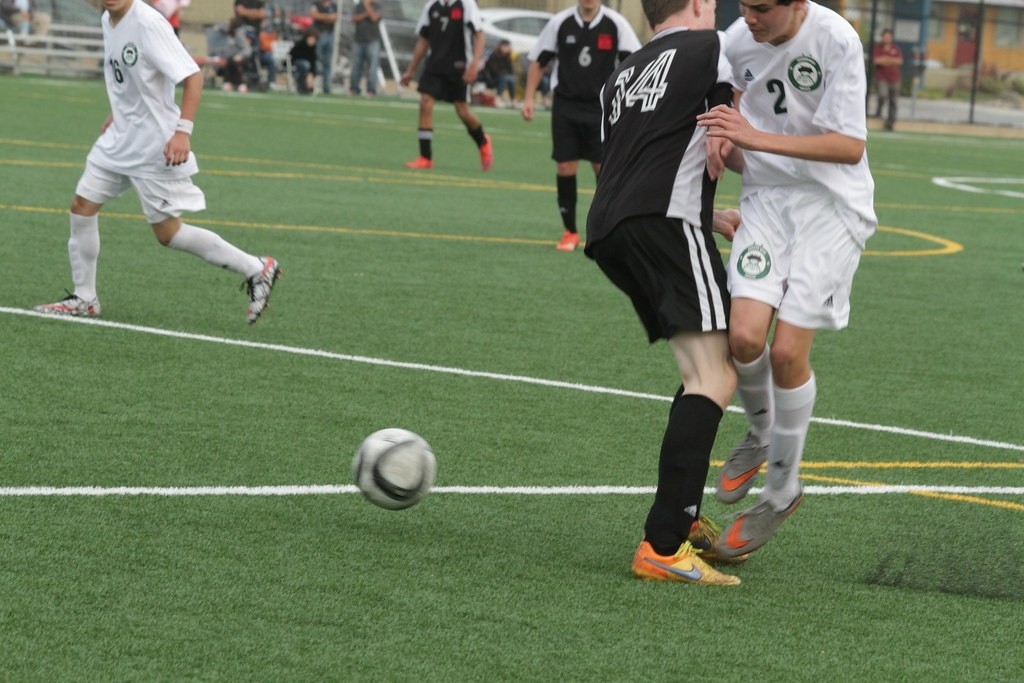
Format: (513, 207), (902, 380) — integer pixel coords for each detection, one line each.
(173, 119), (194, 135)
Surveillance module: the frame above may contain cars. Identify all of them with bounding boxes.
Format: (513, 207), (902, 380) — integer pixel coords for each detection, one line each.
(332, 14), (418, 85)
(477, 5), (559, 57)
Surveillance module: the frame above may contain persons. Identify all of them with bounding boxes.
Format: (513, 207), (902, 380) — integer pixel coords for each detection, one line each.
(871, 28), (904, 131)
(521, 0), (643, 251)
(351, 0), (382, 95)
(399, 0), (494, 171)
(583, 0), (743, 590)
(36, 0), (280, 326)
(486, 39), (518, 109)
(694, 0), (878, 561)
(149, 0), (190, 36)
(284, 32), (317, 93)
(310, 0), (338, 94)
(0, 0), (52, 45)
(205, 0), (278, 95)
(538, 65), (554, 106)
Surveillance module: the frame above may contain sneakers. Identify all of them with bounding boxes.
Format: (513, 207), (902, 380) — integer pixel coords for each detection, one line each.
(406, 158), (434, 170)
(715, 430), (769, 504)
(688, 516), (749, 562)
(631, 539), (740, 587)
(558, 232), (579, 251)
(479, 135), (493, 170)
(238, 257), (283, 325)
(34, 287), (101, 319)
(716, 492), (804, 562)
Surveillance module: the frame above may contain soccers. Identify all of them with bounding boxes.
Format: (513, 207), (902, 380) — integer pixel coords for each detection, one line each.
(352, 428), (437, 511)
